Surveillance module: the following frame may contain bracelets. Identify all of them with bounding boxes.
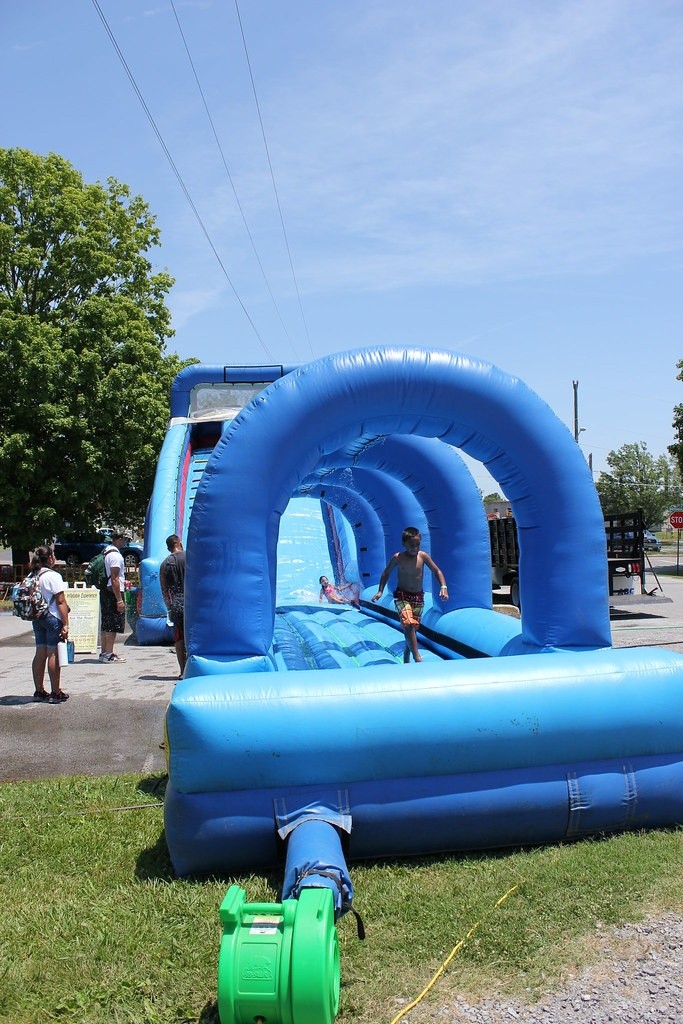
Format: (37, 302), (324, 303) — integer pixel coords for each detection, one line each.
(440, 585), (448, 590)
(117, 600), (123, 604)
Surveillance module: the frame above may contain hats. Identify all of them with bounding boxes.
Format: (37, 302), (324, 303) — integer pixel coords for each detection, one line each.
(112, 528), (128, 539)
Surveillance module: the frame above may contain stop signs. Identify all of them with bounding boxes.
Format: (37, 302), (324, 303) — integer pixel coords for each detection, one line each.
(670, 511), (683, 529)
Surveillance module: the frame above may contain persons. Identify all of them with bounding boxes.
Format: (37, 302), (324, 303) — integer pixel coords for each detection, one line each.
(318, 576), (351, 604)
(29, 547), (69, 705)
(158, 535), (186, 678)
(97, 529), (133, 663)
(371, 527), (449, 664)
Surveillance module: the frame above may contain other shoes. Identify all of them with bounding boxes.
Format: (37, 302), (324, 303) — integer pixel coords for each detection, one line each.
(34, 690), (51, 702)
(49, 688), (69, 703)
(103, 653), (126, 663)
(98, 653), (106, 661)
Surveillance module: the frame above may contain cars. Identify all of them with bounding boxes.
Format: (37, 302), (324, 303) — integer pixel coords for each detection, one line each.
(610, 528), (663, 552)
(52, 526), (144, 567)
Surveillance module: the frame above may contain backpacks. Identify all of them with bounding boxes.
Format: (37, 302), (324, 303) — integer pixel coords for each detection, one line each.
(84, 546), (118, 589)
(12, 569), (55, 621)
